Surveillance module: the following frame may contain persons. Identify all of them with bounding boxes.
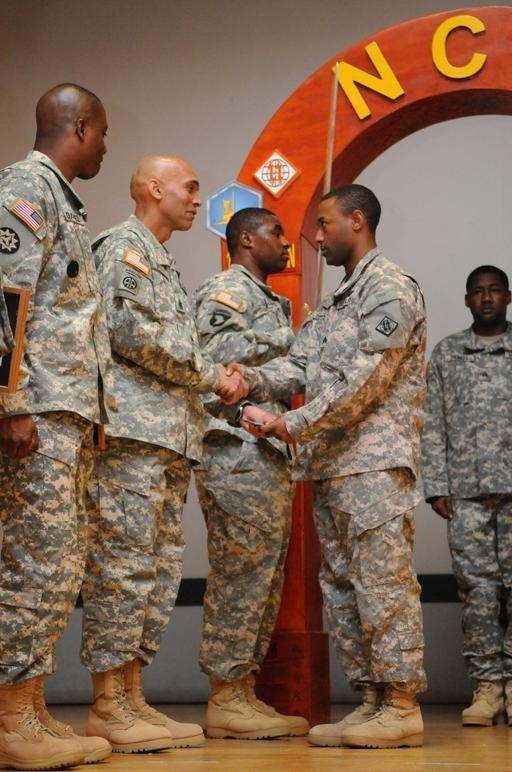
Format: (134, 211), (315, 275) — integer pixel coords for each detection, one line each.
(85, 153), (282, 754)
(424, 260), (512, 727)
(194, 205), (312, 740)
(1, 83), (122, 771)
(209, 182), (431, 748)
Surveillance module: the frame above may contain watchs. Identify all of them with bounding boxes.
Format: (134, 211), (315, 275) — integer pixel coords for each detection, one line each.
(234, 405), (247, 423)
